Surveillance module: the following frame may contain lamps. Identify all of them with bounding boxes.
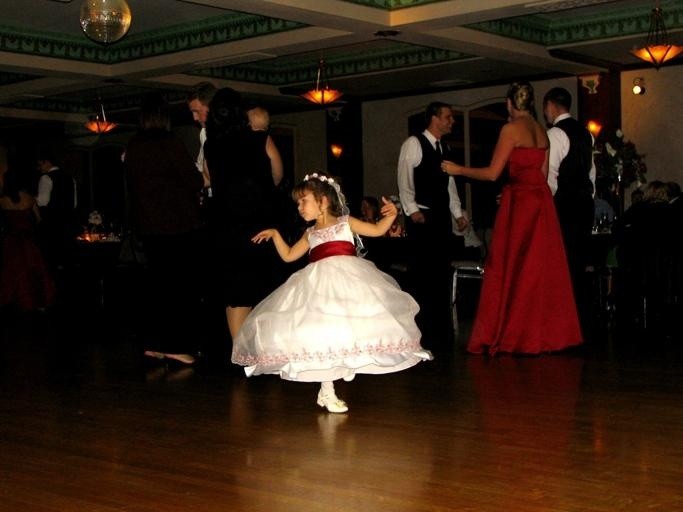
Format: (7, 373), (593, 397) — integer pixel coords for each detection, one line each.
(585, 119), (603, 202)
(633, 77), (643, 95)
(83, 117), (120, 134)
(630, 7), (683, 69)
(300, 58), (344, 105)
(75, 1), (134, 46)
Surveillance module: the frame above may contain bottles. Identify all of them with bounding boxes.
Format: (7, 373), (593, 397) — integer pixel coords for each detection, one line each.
(598, 207), (608, 230)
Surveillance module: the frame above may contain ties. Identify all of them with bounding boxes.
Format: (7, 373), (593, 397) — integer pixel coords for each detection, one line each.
(435, 141), (441, 153)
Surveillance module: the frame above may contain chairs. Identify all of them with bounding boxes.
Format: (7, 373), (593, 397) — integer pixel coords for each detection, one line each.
(450, 228), (494, 333)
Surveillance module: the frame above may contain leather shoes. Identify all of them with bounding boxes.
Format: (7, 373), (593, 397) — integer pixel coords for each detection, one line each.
(316, 392), (348, 413)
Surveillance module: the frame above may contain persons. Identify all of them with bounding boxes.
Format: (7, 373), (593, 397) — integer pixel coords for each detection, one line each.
(543, 87), (598, 257)
(229, 173), (433, 415)
(1, 81), (284, 363)
(396, 105), (469, 351)
(440, 78), (584, 357)
(615, 180), (682, 229)
(358, 196), (402, 237)
